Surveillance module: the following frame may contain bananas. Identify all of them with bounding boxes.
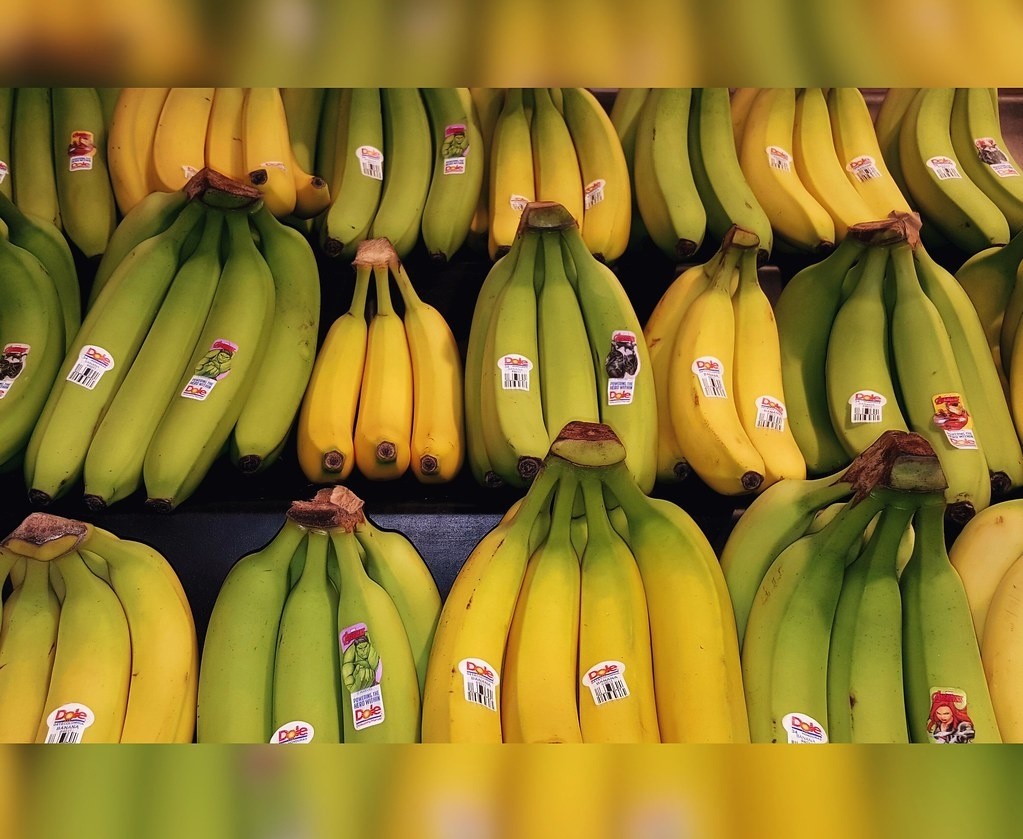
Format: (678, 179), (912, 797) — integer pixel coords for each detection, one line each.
(0, 88), (1023, 744)
